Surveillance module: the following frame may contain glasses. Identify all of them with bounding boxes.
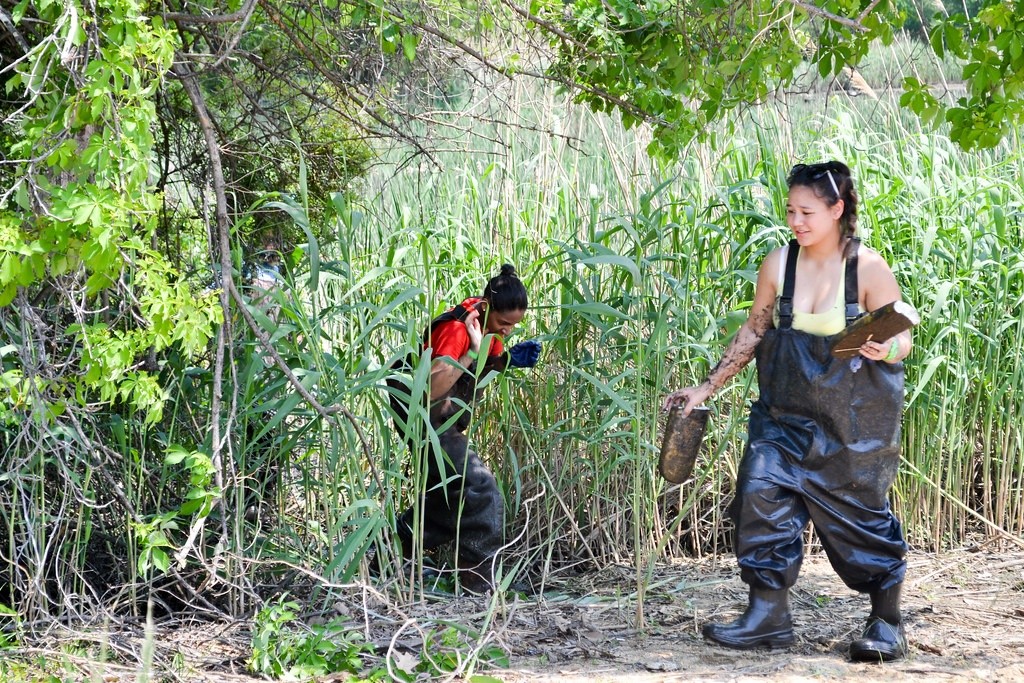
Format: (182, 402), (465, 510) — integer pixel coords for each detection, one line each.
(790, 161), (840, 198)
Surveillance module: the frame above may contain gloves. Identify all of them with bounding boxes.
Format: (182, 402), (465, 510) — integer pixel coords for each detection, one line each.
(500, 339), (542, 372)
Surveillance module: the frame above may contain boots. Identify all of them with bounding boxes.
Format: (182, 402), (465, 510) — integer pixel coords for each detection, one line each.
(698, 583), (801, 645)
(847, 582), (911, 660)
(362, 517), (417, 578)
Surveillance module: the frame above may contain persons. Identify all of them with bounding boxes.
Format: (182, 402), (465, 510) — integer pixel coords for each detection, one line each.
(383, 263), (540, 594)
(662, 160), (920, 661)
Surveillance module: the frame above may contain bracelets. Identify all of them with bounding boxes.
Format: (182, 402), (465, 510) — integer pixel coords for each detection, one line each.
(466, 349), (478, 361)
(882, 339), (898, 362)
